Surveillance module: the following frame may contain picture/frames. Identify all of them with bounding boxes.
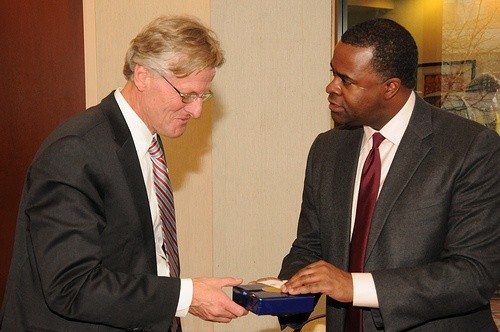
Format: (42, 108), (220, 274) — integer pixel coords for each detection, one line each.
(415, 59), (476, 110)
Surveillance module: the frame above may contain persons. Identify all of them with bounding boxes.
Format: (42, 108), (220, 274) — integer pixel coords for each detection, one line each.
(445, 72), (500, 132)
(249, 17), (500, 332)
(1, 11), (248, 331)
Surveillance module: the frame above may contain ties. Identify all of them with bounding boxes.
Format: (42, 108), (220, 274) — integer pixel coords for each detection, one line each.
(149, 137), (181, 332)
(344, 133), (386, 332)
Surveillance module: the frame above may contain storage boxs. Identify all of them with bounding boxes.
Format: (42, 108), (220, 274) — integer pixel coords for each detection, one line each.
(232, 286), (315, 316)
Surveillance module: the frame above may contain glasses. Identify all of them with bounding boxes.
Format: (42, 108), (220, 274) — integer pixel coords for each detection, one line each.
(152, 70), (215, 104)
